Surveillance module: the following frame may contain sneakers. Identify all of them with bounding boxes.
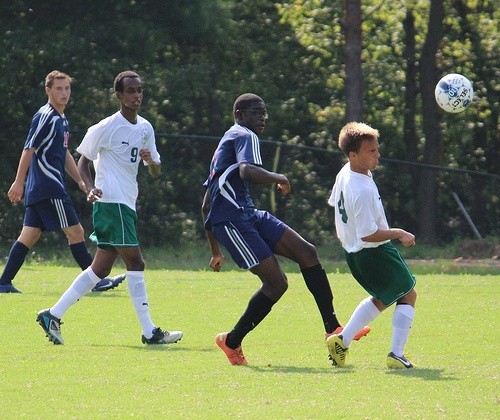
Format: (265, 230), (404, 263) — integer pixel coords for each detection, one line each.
(216, 333), (247, 366)
(141, 327), (183, 345)
(35, 308), (64, 345)
(386, 352), (412, 370)
(92, 273), (126, 293)
(326, 335), (349, 367)
(325, 325), (371, 344)
(0, 285), (23, 295)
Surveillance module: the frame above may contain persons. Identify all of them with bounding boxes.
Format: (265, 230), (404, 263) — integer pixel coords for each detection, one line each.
(35, 71), (184, 346)
(0, 70), (126, 293)
(325, 121), (422, 370)
(203, 93), (373, 366)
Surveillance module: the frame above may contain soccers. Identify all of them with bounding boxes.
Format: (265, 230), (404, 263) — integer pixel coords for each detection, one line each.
(433, 73), (475, 115)
